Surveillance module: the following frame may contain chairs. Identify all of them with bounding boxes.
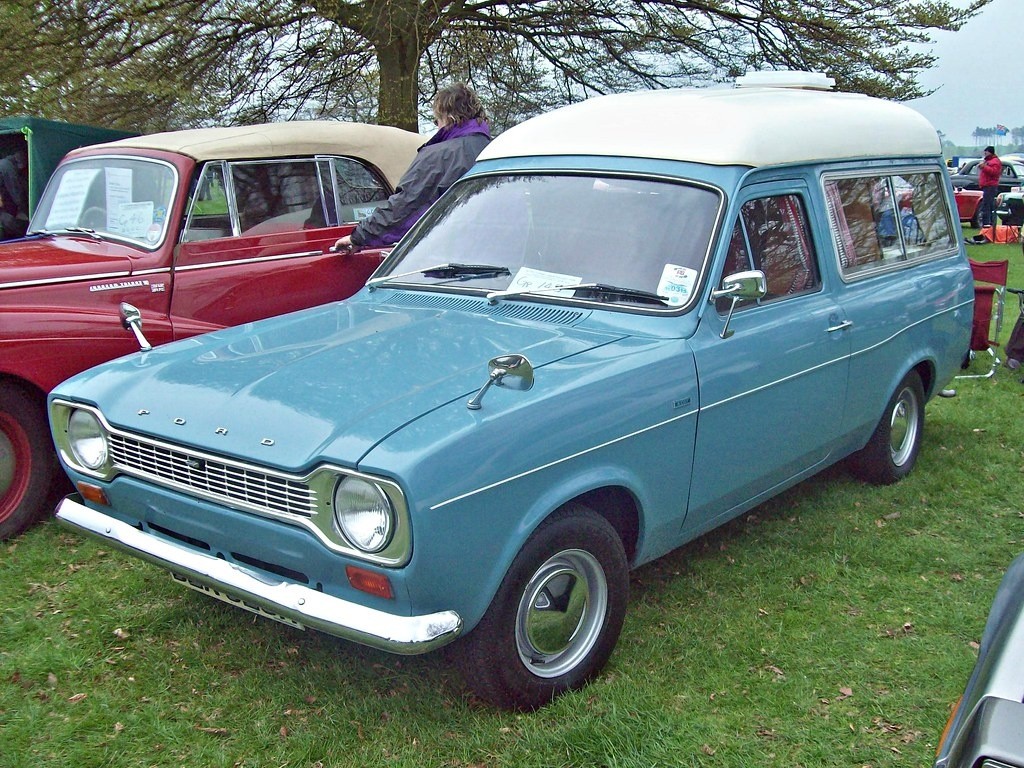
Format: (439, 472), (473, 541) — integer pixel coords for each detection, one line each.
(953, 259), (1024, 379)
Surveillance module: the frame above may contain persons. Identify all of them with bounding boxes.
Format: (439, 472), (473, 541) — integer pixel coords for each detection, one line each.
(335, 82), (495, 255)
(978, 146), (1002, 227)
(303, 162), (371, 230)
(871, 176), (927, 261)
(0, 150), (29, 242)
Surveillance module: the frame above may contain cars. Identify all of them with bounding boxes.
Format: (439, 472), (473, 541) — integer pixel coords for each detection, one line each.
(946, 154), (1024, 229)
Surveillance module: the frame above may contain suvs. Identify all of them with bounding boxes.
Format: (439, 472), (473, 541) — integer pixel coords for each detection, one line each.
(47, 72), (975, 713)
(0, 122), (430, 540)
